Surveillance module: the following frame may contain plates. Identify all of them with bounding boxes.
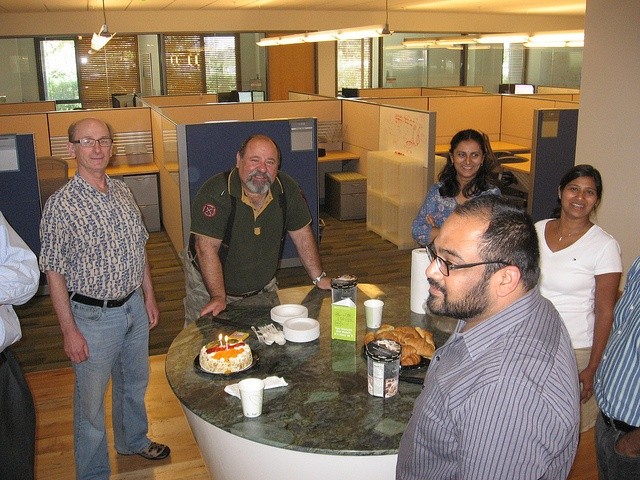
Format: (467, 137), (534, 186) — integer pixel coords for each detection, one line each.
(360, 343), (437, 370)
(193, 349), (260, 375)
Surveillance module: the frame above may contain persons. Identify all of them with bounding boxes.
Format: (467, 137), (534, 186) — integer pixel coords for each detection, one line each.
(0, 213), (42, 480)
(38, 118), (171, 480)
(410, 128), (503, 246)
(533, 165), (623, 480)
(179, 134), (332, 327)
(592, 256), (639, 479)
(396, 195), (582, 478)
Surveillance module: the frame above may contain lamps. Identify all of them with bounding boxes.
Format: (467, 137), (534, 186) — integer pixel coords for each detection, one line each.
(400, 37), (479, 50)
(255, 2), (393, 49)
(89, 11), (114, 52)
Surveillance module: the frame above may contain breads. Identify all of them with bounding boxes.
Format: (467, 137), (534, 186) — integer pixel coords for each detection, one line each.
(364, 324), (436, 367)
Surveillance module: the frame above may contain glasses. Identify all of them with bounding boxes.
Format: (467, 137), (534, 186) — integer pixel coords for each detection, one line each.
(425, 241), (512, 277)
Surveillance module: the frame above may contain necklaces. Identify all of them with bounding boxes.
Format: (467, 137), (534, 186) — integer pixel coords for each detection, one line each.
(555, 224), (583, 241)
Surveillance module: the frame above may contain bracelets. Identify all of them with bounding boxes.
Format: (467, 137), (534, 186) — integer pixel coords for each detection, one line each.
(313, 270), (328, 286)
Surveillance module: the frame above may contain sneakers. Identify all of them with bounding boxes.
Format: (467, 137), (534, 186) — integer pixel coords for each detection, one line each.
(118, 441), (170, 460)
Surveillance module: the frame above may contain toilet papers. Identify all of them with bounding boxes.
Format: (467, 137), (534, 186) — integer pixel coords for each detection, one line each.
(410, 248), (431, 315)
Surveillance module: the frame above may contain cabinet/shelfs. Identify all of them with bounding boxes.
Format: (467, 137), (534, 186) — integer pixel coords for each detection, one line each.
(323, 170), (366, 219)
(367, 148), (427, 250)
(119, 175), (164, 235)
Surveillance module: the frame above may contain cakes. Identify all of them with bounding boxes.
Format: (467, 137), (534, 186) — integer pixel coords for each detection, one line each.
(199, 338), (254, 375)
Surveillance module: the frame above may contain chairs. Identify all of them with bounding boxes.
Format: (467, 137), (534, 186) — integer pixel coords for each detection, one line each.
(36, 157), (66, 208)
(500, 151), (532, 218)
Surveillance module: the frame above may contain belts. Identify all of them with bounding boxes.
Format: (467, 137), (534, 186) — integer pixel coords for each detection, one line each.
(68, 290), (136, 309)
(600, 409), (638, 434)
(187, 245), (275, 298)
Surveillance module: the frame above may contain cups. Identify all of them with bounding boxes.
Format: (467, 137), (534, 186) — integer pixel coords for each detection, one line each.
(237, 378), (266, 418)
(363, 299), (385, 329)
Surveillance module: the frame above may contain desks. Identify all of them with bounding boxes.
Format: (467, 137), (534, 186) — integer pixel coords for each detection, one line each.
(36, 161), (157, 184)
(320, 149), (360, 165)
(436, 139), (530, 194)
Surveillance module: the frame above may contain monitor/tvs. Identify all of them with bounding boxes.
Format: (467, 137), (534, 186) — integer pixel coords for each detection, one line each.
(111, 94), (136, 108)
(217, 91), (252, 102)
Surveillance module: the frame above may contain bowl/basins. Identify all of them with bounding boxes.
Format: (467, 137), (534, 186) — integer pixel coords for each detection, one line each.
(283, 316), (321, 344)
(270, 303), (309, 324)
